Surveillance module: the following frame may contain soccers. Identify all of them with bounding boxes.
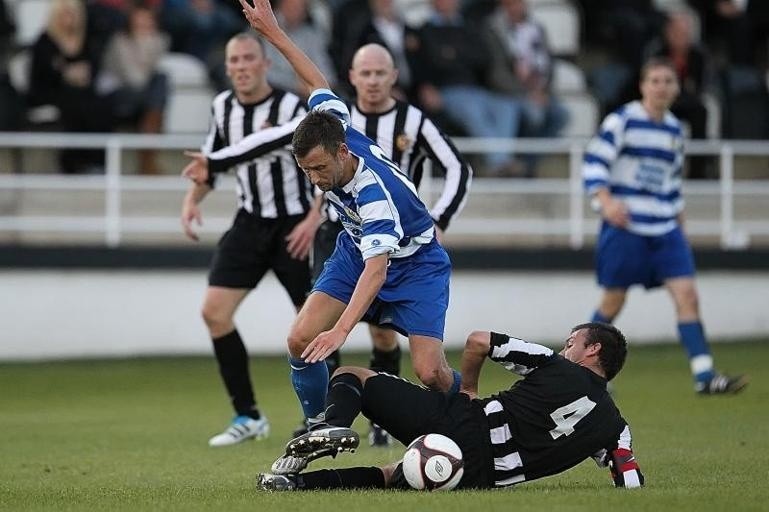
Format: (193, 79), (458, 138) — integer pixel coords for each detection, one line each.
(401, 433), (466, 493)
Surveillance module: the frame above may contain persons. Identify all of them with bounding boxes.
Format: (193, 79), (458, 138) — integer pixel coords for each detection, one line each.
(238, 0), (462, 471)
(256, 323), (646, 491)
(584, 58), (749, 397)
(183, 33), (342, 449)
(308, 44), (472, 447)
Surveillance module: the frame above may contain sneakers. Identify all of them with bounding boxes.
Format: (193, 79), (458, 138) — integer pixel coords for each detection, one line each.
(256, 473), (295, 492)
(209, 416), (269, 447)
(271, 453), (339, 473)
(369, 426), (390, 448)
(286, 426), (360, 454)
(695, 374), (748, 396)
